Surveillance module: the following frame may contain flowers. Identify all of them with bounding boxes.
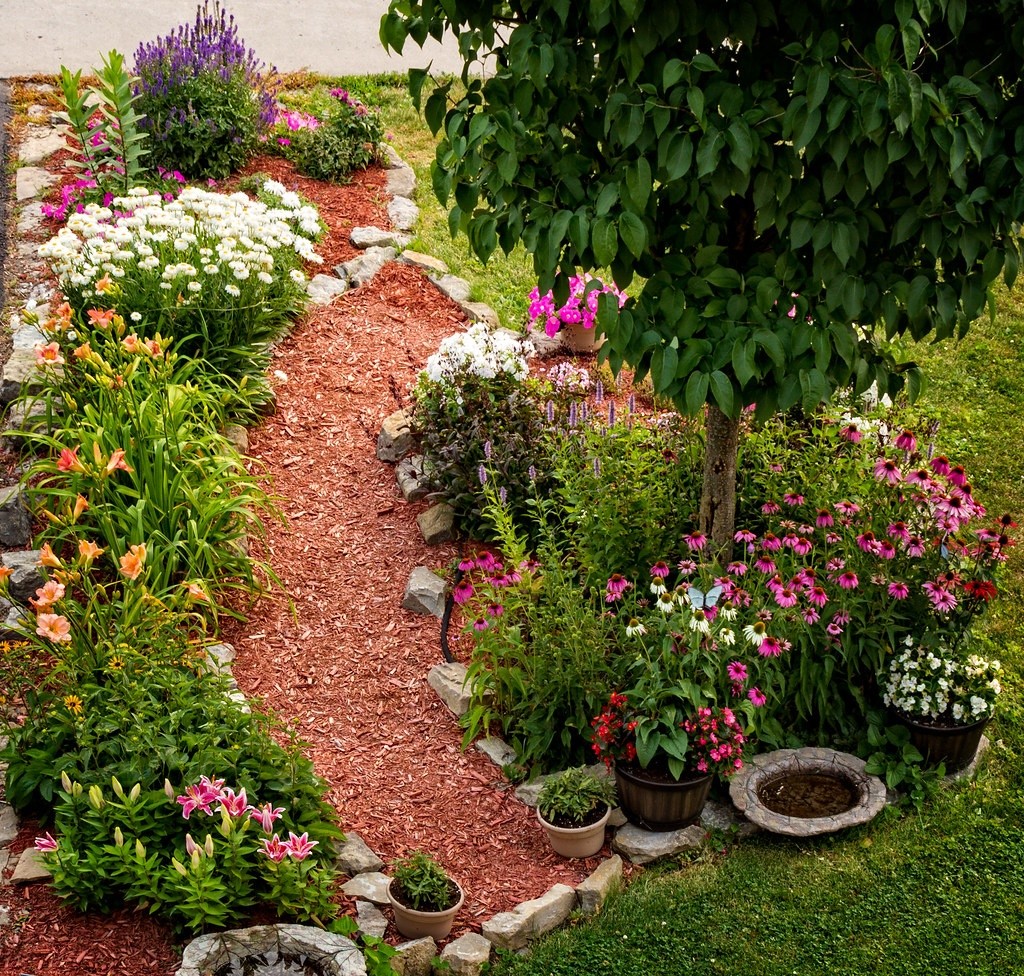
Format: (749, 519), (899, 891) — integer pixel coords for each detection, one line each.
(882, 634), (1005, 728)
(526, 273), (629, 339)
(587, 671), (749, 778)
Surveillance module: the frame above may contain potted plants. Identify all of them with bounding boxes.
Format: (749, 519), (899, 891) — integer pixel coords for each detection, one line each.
(536, 761), (618, 860)
(386, 847), (466, 941)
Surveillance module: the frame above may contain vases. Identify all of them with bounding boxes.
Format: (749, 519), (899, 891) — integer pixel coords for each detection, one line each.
(891, 709), (989, 777)
(559, 324), (607, 353)
(614, 763), (712, 833)
